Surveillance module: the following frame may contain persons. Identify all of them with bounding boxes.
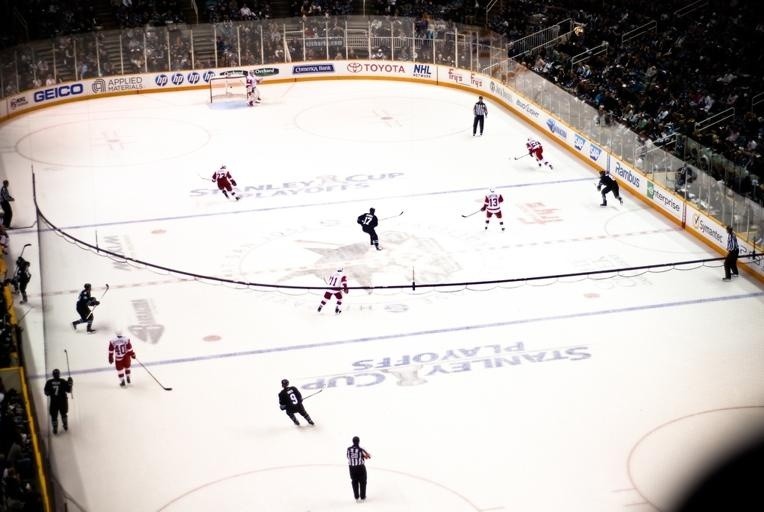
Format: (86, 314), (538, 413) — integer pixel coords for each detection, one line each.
(722, 225), (739, 281)
(195, 1), (359, 69)
(246, 71), (263, 107)
(108, 328), (136, 387)
(317, 265), (349, 313)
(211, 165), (240, 201)
(481, 187), (505, 232)
(11, 256), (32, 304)
(357, 208), (382, 251)
(743, 204), (753, 227)
(2, 180), (15, 230)
(503, 0), (764, 210)
(0, 278), (44, 512)
(367, 1), (509, 69)
(72, 284), (100, 332)
(1, 1), (192, 96)
(346, 436), (370, 500)
(277, 379), (315, 426)
(44, 368), (73, 434)
(472, 96), (487, 136)
